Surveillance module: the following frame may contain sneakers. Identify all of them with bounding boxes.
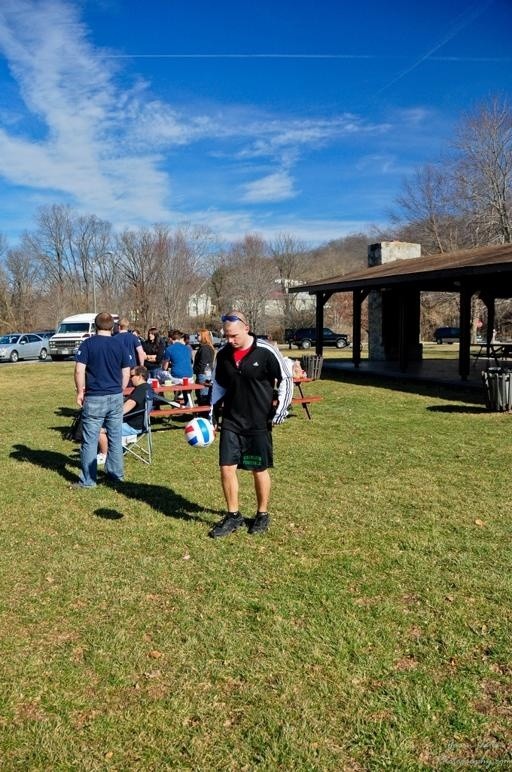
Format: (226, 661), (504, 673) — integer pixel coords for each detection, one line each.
(209, 512), (246, 539)
(248, 514), (270, 535)
(97, 454), (107, 465)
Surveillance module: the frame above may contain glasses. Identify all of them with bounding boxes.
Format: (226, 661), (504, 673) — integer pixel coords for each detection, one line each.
(221, 315), (248, 326)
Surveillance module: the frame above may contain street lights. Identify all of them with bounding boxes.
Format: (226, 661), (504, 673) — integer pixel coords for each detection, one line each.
(92, 250), (112, 313)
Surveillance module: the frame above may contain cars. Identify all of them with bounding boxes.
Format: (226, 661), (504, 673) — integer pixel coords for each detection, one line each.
(189, 330), (272, 350)
(36, 332), (55, 341)
(0, 333), (49, 362)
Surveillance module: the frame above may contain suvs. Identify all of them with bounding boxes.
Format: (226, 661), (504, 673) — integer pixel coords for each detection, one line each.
(293, 327), (351, 349)
(432, 327), (461, 344)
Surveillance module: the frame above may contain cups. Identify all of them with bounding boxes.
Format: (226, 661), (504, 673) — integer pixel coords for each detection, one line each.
(299, 370), (304, 378)
(183, 377), (188, 386)
(198, 374), (205, 384)
(152, 379), (158, 389)
(165, 381), (172, 385)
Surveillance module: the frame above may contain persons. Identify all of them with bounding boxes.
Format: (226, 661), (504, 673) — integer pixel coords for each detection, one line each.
(72, 312), (214, 489)
(208, 311), (293, 538)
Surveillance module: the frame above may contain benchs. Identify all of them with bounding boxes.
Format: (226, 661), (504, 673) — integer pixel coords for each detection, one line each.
(149, 406), (213, 425)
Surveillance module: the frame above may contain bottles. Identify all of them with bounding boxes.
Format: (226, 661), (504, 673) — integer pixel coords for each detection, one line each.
(204, 363), (212, 379)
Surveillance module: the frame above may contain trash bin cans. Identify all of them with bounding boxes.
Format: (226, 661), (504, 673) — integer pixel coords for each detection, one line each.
(302, 354), (323, 381)
(481, 367), (512, 412)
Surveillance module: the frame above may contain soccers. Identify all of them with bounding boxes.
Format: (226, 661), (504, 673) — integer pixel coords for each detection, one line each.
(186, 418), (215, 449)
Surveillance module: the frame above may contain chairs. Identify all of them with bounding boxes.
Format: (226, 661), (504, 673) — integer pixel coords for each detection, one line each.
(122, 389), (154, 464)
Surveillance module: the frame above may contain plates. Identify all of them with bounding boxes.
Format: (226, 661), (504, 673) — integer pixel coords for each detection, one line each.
(162, 384), (175, 386)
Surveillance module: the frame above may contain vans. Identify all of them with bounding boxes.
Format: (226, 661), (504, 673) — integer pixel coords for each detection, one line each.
(48, 313), (119, 360)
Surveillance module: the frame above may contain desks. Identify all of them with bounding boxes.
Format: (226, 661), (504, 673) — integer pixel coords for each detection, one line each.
(471, 344), (512, 367)
(75, 377), (211, 441)
(204, 377), (320, 420)
